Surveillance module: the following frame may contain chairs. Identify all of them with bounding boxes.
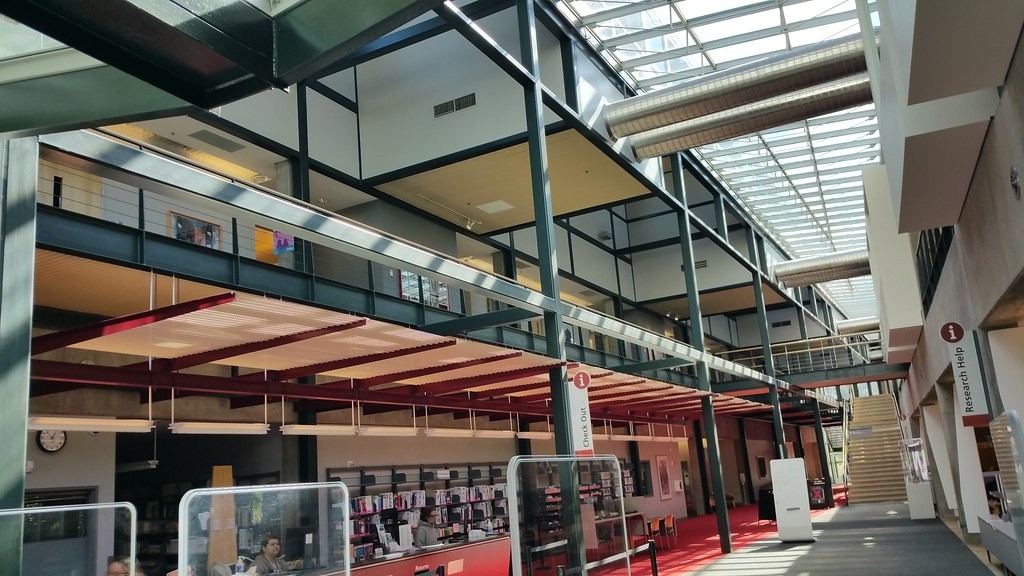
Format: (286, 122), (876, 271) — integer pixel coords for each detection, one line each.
(659, 515), (677, 551)
(726, 493), (736, 508)
(648, 516), (664, 554)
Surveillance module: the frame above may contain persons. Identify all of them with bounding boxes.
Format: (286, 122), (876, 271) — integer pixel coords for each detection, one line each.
(247, 536), (287, 574)
(108, 556), (144, 576)
(415, 506), (441, 546)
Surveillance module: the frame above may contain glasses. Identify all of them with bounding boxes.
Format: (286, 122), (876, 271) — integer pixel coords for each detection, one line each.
(429, 514), (437, 518)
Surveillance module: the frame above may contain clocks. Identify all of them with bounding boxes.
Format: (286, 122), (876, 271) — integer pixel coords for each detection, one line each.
(36, 430), (67, 453)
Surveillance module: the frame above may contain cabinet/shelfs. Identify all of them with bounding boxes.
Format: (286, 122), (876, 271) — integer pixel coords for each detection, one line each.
(325, 461), (528, 567)
(537, 458), (636, 554)
(113, 470), (283, 576)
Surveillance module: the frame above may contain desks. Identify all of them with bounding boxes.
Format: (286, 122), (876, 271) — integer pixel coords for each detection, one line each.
(596, 512), (646, 570)
(648, 516), (677, 537)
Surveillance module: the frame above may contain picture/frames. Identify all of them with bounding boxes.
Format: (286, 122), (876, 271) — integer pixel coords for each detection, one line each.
(656, 456), (672, 500)
(166, 210), (222, 251)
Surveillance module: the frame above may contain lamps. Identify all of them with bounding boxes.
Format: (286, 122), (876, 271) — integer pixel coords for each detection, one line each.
(666, 312), (671, 317)
(516, 399), (553, 440)
(651, 415), (673, 442)
(423, 389), (475, 438)
(607, 410), (634, 441)
(675, 315), (679, 321)
(466, 220), (475, 230)
(279, 377), (358, 435)
(590, 411), (610, 440)
(167, 368), (271, 435)
(357, 400), (420, 437)
(669, 417), (690, 442)
(630, 412), (654, 442)
(740, 473), (750, 506)
(28, 354), (156, 434)
(473, 394), (517, 438)
